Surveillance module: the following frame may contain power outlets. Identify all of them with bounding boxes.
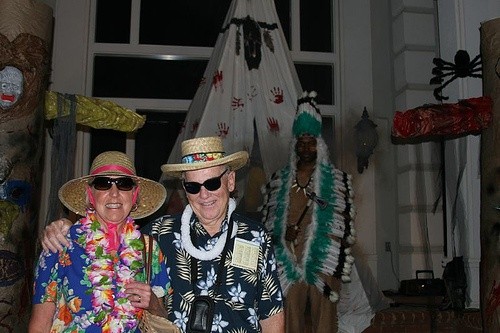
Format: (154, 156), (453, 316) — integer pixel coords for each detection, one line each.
(385, 242), (391, 252)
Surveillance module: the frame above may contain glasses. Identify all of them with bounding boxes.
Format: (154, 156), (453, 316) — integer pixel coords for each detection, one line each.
(89, 176), (138, 192)
(183, 169), (229, 194)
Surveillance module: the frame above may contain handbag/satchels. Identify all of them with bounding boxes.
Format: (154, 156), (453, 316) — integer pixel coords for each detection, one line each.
(185, 296), (215, 333)
(136, 233), (182, 333)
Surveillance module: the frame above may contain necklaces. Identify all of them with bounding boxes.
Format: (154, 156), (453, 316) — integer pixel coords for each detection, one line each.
(180, 197), (239, 261)
(291, 169), (315, 197)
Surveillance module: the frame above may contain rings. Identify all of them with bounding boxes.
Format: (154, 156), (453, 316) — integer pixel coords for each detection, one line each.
(139, 296), (141, 301)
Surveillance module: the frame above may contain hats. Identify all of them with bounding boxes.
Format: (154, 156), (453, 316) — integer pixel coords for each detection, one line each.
(160, 136), (249, 177)
(57, 151), (167, 220)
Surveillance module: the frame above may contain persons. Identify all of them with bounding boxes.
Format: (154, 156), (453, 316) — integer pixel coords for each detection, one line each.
(41, 136), (285, 333)
(28, 151), (171, 333)
(257, 90), (357, 333)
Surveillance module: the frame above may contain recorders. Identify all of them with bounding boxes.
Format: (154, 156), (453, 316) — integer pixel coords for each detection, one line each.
(397, 270), (445, 296)
